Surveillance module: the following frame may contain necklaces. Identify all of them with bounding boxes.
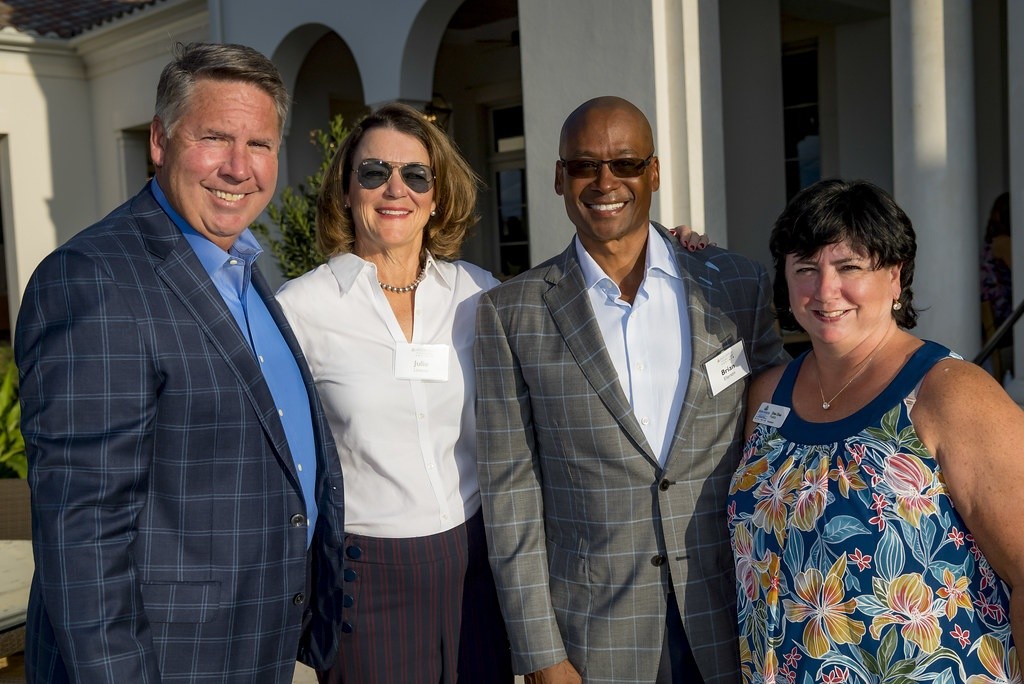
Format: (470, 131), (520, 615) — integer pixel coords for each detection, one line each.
(378, 262), (425, 293)
(816, 354), (872, 410)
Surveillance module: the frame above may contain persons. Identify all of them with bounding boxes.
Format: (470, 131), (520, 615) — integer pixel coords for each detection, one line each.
(474, 98), (795, 684)
(268, 101), (711, 684)
(723, 178), (1024, 684)
(13, 40), (353, 684)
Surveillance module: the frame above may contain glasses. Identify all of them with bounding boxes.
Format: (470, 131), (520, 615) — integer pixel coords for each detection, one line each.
(561, 152), (653, 179)
(352, 158), (436, 194)
